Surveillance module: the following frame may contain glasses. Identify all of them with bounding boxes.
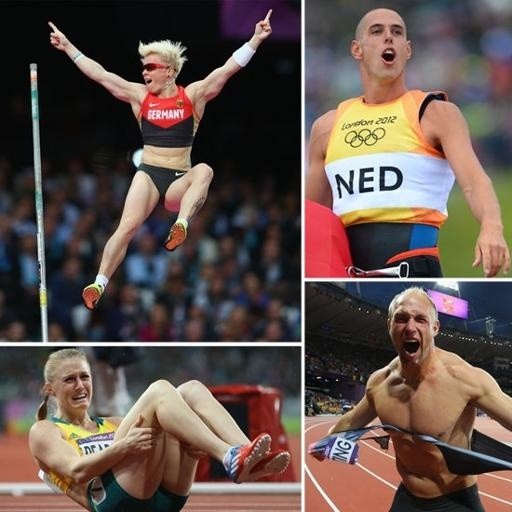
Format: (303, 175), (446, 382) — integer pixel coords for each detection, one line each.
(144, 63), (169, 72)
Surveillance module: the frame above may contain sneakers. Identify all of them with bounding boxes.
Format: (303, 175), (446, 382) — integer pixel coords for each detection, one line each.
(246, 449), (290, 482)
(225, 434), (271, 483)
(82, 281), (103, 311)
(162, 220), (187, 250)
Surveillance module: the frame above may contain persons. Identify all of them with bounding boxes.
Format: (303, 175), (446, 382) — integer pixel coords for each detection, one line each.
(28, 348), (291, 512)
(2, 167), (302, 343)
(304, 340), (377, 415)
(47, 10), (273, 309)
(308, 286), (512, 512)
(306, 9), (512, 278)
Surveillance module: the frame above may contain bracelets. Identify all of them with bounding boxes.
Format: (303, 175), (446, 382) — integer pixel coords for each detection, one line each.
(72, 53), (85, 63)
(232, 41), (256, 68)
